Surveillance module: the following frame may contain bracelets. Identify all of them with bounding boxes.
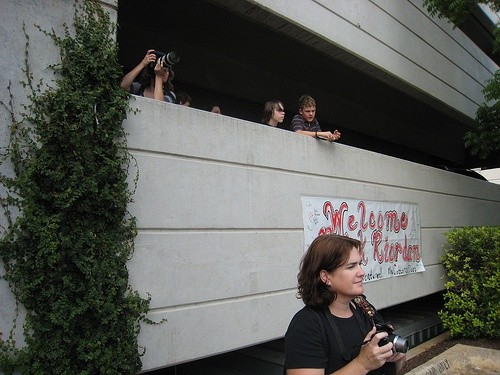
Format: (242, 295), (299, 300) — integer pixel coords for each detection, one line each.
(315, 130), (318, 138)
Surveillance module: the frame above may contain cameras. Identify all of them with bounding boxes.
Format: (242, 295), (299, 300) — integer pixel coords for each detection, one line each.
(371, 322), (409, 354)
(146, 51), (180, 72)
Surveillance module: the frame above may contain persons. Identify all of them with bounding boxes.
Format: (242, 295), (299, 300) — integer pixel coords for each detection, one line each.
(261, 95), (341, 141)
(284, 234), (406, 375)
(120, 44), (193, 107)
